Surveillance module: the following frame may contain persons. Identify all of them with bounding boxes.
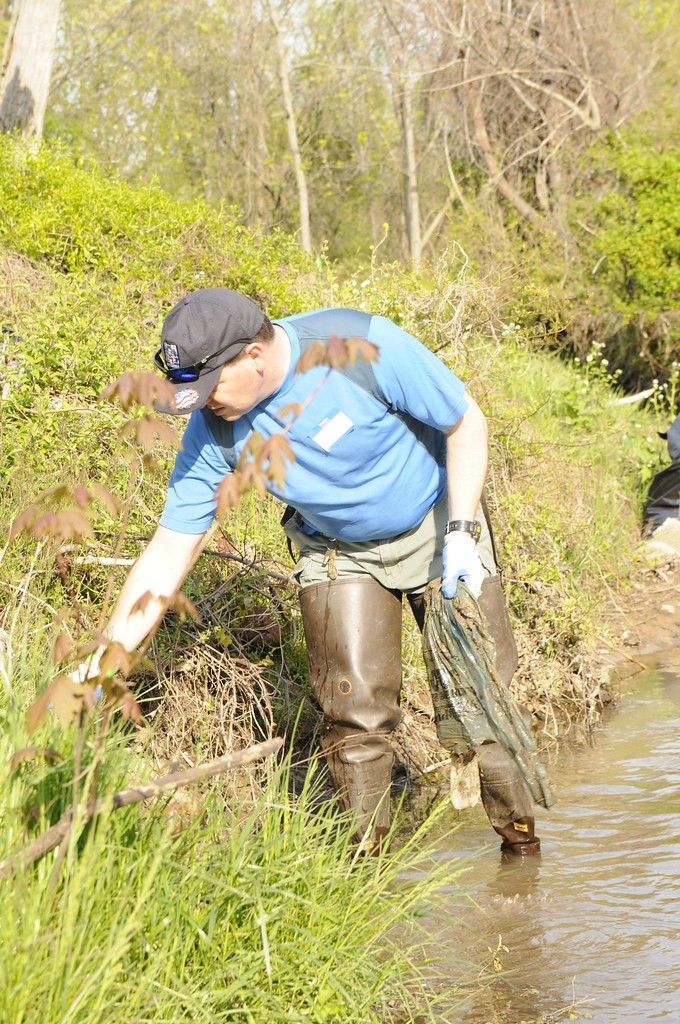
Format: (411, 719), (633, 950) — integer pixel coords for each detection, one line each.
(65, 287), (539, 859)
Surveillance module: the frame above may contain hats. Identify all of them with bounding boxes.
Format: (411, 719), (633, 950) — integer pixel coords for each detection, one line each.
(153, 289), (264, 414)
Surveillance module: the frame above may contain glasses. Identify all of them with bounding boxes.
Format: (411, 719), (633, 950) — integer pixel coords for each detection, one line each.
(154, 339), (255, 382)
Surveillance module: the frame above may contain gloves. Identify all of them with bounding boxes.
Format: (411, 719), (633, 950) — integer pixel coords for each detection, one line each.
(46, 653), (103, 712)
(441, 530), (484, 601)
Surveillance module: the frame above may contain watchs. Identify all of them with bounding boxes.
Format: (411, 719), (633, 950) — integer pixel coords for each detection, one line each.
(445, 520), (481, 546)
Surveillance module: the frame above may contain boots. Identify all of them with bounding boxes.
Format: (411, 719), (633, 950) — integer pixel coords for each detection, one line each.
(406, 574), (540, 857)
(297, 579), (402, 870)
